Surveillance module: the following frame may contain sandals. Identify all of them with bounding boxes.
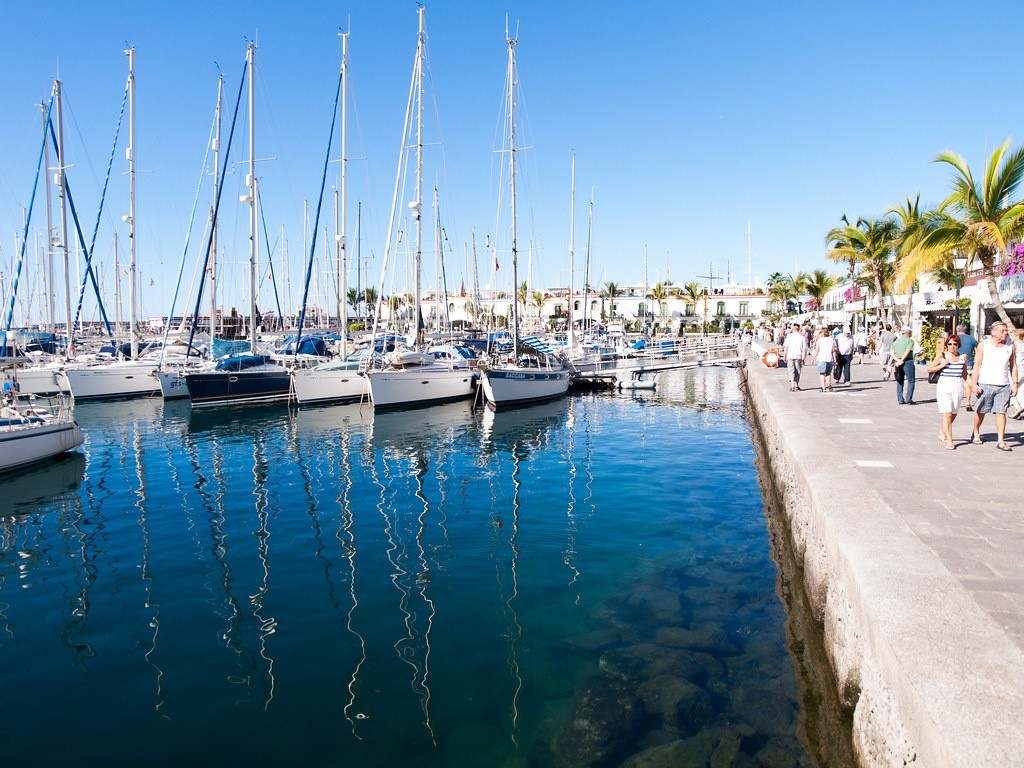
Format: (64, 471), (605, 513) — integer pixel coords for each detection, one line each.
(996, 442), (1012, 451)
(972, 438), (983, 443)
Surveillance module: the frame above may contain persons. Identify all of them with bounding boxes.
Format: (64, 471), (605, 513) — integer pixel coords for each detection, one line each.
(1007, 328), (1024, 420)
(927, 333), (967, 449)
(737, 318), (915, 406)
(969, 321), (1018, 452)
(955, 323), (978, 412)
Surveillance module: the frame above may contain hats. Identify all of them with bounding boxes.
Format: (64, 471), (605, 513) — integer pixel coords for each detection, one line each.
(843, 328), (850, 333)
(900, 327), (913, 333)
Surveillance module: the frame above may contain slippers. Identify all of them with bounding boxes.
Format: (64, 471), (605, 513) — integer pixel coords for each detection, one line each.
(938, 436), (947, 442)
(944, 440), (955, 450)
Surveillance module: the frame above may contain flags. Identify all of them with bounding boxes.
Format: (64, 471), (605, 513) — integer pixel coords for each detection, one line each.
(495, 258), (499, 272)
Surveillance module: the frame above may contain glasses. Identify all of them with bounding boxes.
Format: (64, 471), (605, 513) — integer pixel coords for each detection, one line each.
(947, 343), (958, 347)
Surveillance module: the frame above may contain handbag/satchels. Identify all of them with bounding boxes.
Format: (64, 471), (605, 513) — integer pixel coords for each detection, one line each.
(928, 363), (941, 384)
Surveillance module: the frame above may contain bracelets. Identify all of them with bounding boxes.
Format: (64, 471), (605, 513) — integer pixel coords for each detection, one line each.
(1012, 382), (1018, 384)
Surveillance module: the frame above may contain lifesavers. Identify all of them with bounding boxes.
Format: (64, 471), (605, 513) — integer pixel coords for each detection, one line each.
(762, 349), (782, 368)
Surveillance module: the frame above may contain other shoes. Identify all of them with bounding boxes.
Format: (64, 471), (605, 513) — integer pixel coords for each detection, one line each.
(834, 379), (839, 383)
(966, 404), (973, 411)
(789, 386), (800, 392)
(1008, 408), (1024, 420)
(883, 371), (892, 381)
(859, 360), (863, 365)
(843, 381), (851, 386)
(827, 387), (833, 392)
(898, 402), (907, 405)
(819, 389), (826, 393)
(905, 400), (917, 405)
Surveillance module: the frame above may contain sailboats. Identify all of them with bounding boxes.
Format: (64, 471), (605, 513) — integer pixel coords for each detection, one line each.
(0, 18), (680, 477)
(1, 451), (86, 649)
